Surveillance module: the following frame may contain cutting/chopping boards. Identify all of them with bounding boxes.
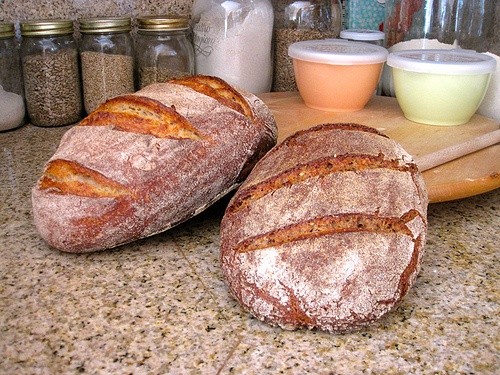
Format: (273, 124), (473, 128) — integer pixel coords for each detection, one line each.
(252, 91), (500, 202)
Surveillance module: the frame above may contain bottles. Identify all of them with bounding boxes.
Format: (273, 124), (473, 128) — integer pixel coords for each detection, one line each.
(20, 20), (84, 127)
(135, 16), (194, 90)
(340, 0), (500, 58)
(193, 0), (275, 93)
(271, 0), (342, 93)
(0, 22), (27, 133)
(78, 17), (136, 114)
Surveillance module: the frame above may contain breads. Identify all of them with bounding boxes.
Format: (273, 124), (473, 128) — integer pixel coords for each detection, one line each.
(31, 75), (278, 253)
(221, 122), (429, 332)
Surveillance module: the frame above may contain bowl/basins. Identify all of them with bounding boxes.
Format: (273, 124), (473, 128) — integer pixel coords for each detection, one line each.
(387, 50), (497, 126)
(288, 39), (390, 113)
